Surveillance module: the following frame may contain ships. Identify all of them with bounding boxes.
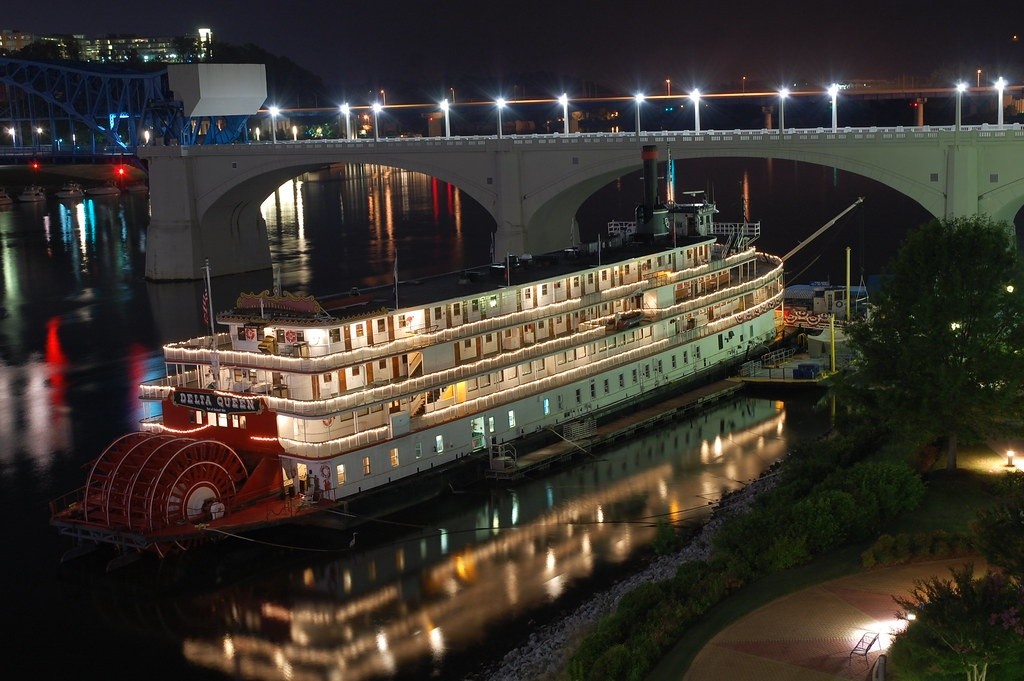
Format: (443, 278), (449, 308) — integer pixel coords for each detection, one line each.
(48, 142), (868, 562)
(184, 398), (824, 681)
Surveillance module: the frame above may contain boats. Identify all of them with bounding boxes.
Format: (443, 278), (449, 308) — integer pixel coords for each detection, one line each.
(89, 179), (120, 195)
(19, 184), (47, 201)
(55, 181), (87, 198)
(0, 186), (14, 205)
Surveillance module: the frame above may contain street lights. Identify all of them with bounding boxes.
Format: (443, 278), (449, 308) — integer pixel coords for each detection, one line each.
(0, 73), (1017, 149)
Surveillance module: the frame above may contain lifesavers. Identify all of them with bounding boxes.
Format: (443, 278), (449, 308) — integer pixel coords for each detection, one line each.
(323, 417), (333, 427)
(320, 465), (330, 478)
(286, 330), (296, 342)
(246, 329), (255, 339)
